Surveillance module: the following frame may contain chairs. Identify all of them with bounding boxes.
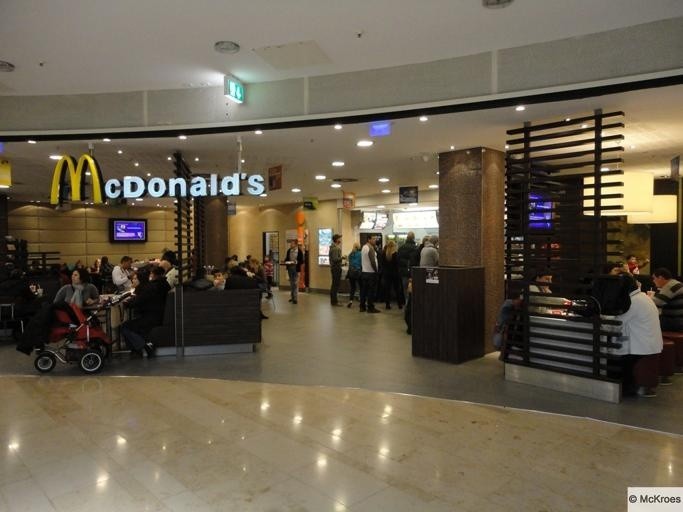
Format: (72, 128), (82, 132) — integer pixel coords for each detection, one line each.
(0, 296), (25, 339)
(85, 274), (124, 295)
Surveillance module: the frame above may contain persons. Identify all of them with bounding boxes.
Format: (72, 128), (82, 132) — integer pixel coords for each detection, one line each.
(493, 255), (683, 398)
(283, 239), (304, 304)
(0, 245), (180, 359)
(207, 254), (275, 299)
(329, 228), (440, 335)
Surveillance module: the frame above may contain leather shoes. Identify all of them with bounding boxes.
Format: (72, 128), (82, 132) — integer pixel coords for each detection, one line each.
(145, 342), (156, 359)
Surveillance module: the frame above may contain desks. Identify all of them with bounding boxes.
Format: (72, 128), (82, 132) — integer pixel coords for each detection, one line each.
(82, 293), (136, 355)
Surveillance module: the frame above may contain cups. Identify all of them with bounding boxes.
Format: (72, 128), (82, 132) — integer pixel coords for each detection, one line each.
(29, 285), (43, 295)
(646, 290), (655, 297)
(143, 256), (149, 263)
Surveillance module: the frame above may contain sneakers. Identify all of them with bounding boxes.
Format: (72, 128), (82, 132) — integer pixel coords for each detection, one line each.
(289, 297), (403, 313)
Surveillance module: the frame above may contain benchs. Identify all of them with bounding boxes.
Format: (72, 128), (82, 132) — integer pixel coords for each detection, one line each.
(183, 288), (262, 356)
(142, 287), (178, 358)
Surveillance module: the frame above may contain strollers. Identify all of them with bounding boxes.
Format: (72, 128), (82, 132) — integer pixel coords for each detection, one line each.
(31, 291), (132, 372)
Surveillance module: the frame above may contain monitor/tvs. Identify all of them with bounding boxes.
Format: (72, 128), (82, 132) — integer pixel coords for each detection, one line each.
(108, 217), (148, 243)
(528, 191), (553, 231)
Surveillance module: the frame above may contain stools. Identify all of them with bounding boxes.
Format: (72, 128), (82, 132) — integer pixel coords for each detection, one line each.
(632, 331), (683, 398)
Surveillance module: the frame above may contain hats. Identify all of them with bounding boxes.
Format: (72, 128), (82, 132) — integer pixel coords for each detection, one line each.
(332, 234), (342, 242)
